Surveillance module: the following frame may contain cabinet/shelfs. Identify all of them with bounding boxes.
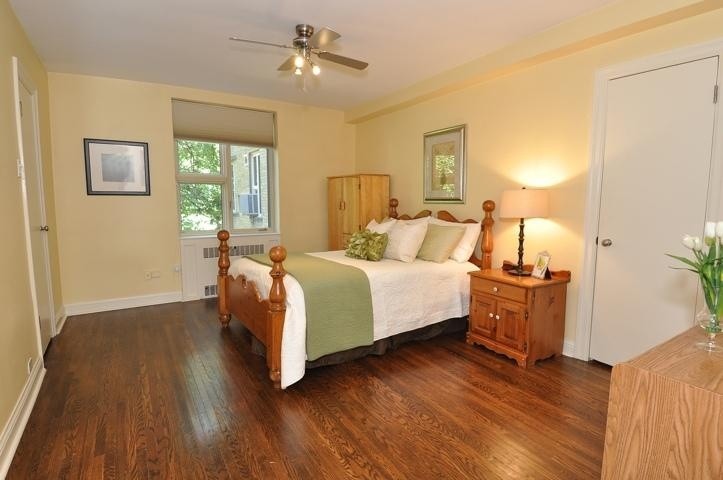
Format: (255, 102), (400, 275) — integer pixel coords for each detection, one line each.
(327, 173), (390, 253)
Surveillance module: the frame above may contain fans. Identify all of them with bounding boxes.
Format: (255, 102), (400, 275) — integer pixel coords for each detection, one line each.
(224, 23), (370, 71)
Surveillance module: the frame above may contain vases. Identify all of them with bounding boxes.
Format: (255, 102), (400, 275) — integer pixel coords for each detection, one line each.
(695, 288), (722, 354)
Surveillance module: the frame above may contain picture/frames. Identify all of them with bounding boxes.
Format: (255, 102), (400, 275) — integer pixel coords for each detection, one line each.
(82, 137), (152, 197)
(421, 123), (468, 204)
(528, 254), (553, 279)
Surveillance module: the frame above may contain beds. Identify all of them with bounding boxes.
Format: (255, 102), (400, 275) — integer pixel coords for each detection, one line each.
(215, 196), (496, 394)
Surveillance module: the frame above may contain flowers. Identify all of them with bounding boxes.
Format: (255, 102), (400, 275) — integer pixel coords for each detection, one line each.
(666, 216), (723, 298)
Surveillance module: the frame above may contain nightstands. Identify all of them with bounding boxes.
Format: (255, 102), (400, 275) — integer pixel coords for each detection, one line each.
(466, 263), (572, 371)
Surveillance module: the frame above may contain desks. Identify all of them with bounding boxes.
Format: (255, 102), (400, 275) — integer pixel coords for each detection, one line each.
(598, 316), (723, 480)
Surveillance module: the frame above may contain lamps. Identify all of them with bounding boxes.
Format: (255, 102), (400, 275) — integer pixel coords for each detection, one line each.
(496, 188), (555, 276)
(293, 48), (320, 76)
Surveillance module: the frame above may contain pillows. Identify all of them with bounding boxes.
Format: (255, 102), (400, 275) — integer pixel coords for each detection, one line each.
(344, 214), (482, 264)
